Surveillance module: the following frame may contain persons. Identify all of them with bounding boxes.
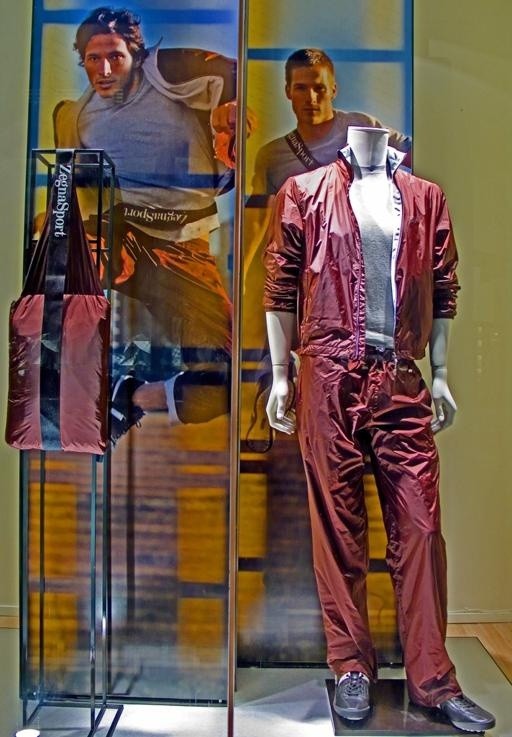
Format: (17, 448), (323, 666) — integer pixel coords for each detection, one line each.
(35, 8), (255, 424)
(260, 125), (495, 731)
(239, 48), (412, 297)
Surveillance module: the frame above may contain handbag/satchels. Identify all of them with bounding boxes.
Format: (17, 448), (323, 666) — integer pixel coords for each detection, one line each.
(5, 295), (111, 455)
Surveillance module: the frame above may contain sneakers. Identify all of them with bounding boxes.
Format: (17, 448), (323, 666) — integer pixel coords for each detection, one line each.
(437, 694), (495, 733)
(96, 375), (146, 462)
(333, 671), (370, 720)
